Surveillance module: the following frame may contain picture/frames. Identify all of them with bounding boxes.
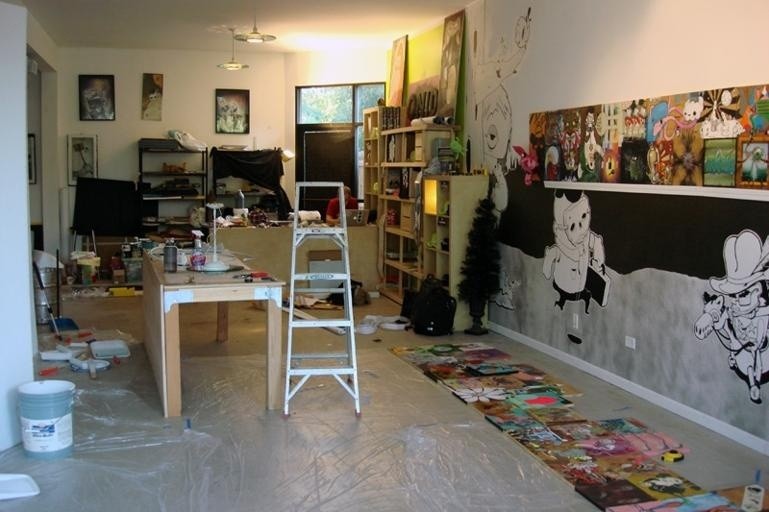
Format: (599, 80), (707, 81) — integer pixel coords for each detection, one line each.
(215, 88), (250, 134)
(79, 74), (115, 120)
(141, 72), (164, 121)
(386, 35), (406, 105)
(29, 132), (37, 188)
(67, 133), (96, 185)
(437, 10), (465, 125)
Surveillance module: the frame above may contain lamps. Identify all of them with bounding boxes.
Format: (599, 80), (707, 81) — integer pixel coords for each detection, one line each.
(232, 27), (276, 44)
(216, 57), (249, 71)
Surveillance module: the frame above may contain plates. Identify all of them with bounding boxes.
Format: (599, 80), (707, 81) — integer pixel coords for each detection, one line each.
(90, 340), (131, 359)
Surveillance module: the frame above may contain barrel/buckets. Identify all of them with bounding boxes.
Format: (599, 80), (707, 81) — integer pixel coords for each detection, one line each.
(16, 379), (76, 461)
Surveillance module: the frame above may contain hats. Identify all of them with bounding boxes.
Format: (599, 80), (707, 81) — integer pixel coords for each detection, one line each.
(709, 228), (769, 295)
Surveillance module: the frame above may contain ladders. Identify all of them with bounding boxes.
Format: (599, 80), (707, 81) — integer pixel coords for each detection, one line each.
(284, 182), (362, 419)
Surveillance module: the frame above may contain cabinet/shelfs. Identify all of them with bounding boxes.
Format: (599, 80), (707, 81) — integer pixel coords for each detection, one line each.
(377, 127), (460, 313)
(139, 137), (207, 240)
(362, 105), (403, 222)
(423, 174), (491, 334)
(210, 146), (283, 218)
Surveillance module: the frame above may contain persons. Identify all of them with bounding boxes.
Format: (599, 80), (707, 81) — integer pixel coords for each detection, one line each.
(325, 186), (359, 225)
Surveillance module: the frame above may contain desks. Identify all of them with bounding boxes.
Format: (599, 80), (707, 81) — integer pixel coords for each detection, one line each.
(209, 223), (384, 297)
(142, 247), (287, 419)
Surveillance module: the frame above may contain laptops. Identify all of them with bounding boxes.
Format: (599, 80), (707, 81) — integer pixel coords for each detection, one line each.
(337, 210), (369, 228)
